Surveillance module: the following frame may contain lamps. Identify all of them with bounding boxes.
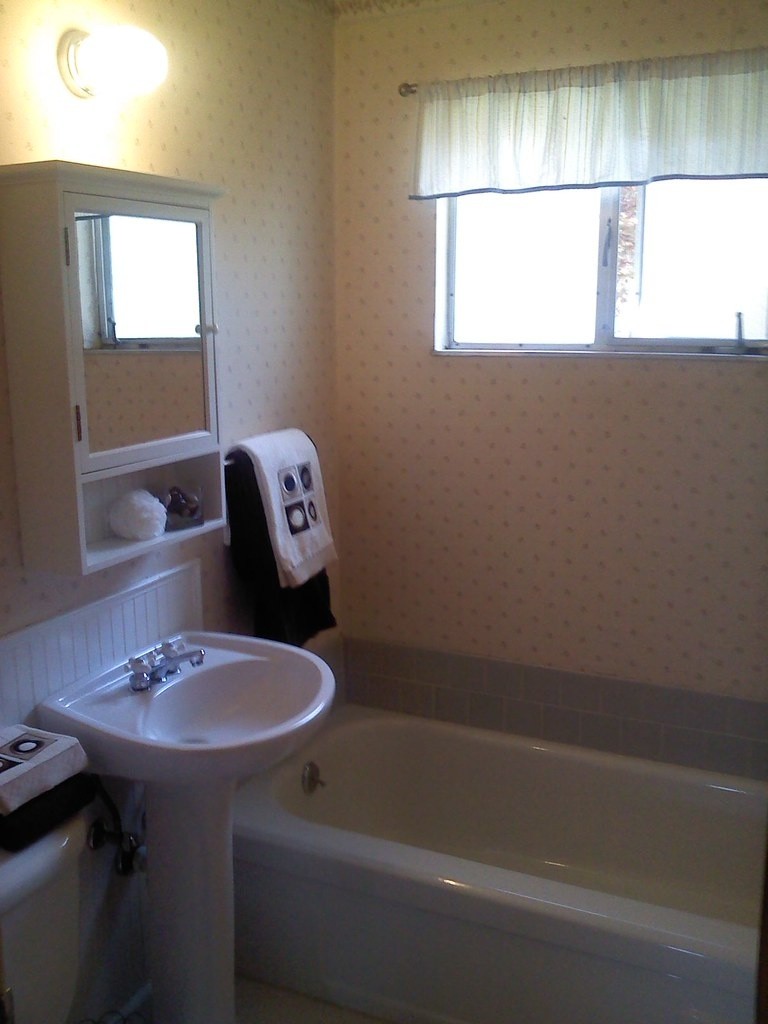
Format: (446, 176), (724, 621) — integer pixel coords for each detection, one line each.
(55, 23), (170, 101)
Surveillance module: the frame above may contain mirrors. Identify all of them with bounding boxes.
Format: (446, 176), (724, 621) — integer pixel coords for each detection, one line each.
(72, 209), (206, 454)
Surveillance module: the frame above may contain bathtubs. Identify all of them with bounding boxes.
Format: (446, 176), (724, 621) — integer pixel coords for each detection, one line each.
(230, 707), (768, 1023)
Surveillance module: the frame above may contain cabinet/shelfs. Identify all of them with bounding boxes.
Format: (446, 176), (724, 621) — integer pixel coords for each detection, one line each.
(0, 160), (229, 578)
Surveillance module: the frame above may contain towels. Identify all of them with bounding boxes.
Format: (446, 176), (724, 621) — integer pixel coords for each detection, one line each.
(223, 426), (339, 589)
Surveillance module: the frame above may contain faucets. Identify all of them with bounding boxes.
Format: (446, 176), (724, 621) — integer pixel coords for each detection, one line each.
(150, 648), (207, 684)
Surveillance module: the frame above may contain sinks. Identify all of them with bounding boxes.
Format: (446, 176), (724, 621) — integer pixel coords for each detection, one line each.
(38, 628), (338, 786)
